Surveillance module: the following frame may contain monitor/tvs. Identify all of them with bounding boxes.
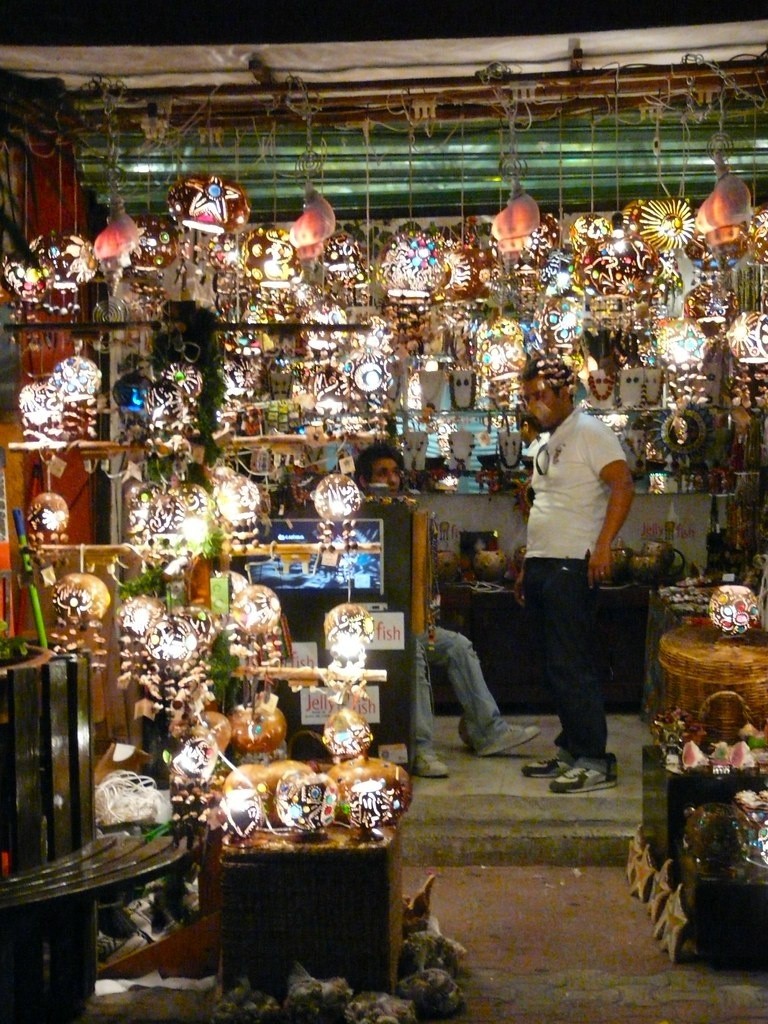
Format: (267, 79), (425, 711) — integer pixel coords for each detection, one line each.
(243, 518), (385, 597)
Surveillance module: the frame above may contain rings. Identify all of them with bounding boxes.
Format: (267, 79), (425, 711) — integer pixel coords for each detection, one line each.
(600, 572), (605, 575)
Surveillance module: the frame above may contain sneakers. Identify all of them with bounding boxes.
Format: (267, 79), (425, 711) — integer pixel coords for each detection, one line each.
(549, 751), (617, 792)
(475, 723), (541, 757)
(521, 747), (578, 778)
(412, 754), (450, 777)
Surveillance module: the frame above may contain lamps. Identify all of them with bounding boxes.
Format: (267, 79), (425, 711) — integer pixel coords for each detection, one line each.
(0, 68), (768, 840)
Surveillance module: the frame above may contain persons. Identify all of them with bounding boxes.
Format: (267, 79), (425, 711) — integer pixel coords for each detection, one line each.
(515, 403), (551, 457)
(353, 440), (541, 779)
(513, 354), (636, 793)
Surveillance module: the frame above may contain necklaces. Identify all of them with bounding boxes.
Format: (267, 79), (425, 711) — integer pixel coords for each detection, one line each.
(269, 365), (667, 475)
